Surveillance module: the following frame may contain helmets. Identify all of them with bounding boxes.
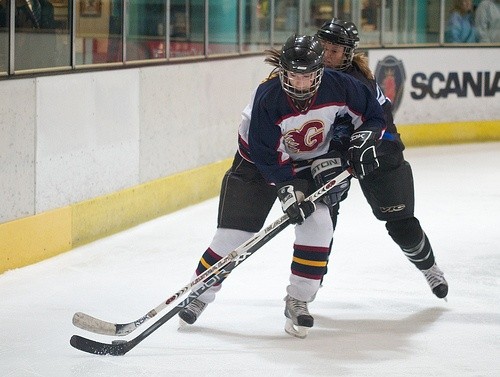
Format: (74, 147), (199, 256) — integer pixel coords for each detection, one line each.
(278, 34), (324, 101)
(316, 18), (359, 70)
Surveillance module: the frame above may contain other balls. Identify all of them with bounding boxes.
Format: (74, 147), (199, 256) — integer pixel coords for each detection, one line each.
(111, 340), (127, 345)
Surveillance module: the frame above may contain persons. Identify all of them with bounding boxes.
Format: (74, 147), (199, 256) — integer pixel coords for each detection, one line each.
(445, 0), (500, 44)
(176, 18), (376, 330)
(247, 35), (449, 338)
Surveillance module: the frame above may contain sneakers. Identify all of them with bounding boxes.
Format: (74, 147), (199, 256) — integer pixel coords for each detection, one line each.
(178, 289), (208, 330)
(423, 262), (448, 302)
(283, 296), (314, 339)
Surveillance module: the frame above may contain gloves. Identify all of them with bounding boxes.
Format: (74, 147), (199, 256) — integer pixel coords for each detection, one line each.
(275, 179), (316, 224)
(310, 151), (349, 205)
(346, 119), (384, 178)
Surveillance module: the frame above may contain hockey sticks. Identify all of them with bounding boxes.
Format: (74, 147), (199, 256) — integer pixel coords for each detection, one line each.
(72, 165), (355, 337)
(69, 220), (289, 356)
